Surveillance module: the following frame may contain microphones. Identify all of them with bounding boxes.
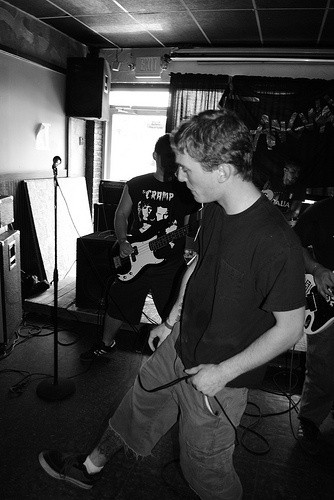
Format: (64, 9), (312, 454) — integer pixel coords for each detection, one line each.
(52, 156), (62, 170)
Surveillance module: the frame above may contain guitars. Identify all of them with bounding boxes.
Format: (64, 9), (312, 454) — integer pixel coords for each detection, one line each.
(303, 270), (334, 335)
(109, 189), (274, 283)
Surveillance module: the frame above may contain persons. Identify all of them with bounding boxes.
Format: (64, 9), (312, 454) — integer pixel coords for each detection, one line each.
(292, 183), (334, 445)
(92, 135), (201, 361)
(140, 202), (156, 223)
(40, 110), (310, 500)
(156, 203), (171, 222)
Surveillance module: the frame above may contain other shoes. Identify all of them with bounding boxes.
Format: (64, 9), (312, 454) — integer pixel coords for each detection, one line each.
(297, 420), (321, 453)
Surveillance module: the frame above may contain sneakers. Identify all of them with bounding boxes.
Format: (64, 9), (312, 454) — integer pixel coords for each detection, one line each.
(81, 339), (117, 361)
(38, 450), (100, 489)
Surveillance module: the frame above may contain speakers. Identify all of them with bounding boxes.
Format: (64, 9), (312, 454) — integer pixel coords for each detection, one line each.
(76, 204), (133, 310)
(0, 229), (22, 346)
(65, 57), (111, 122)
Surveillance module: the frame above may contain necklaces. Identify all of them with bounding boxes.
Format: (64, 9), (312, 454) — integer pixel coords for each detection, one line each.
(259, 165), (303, 228)
